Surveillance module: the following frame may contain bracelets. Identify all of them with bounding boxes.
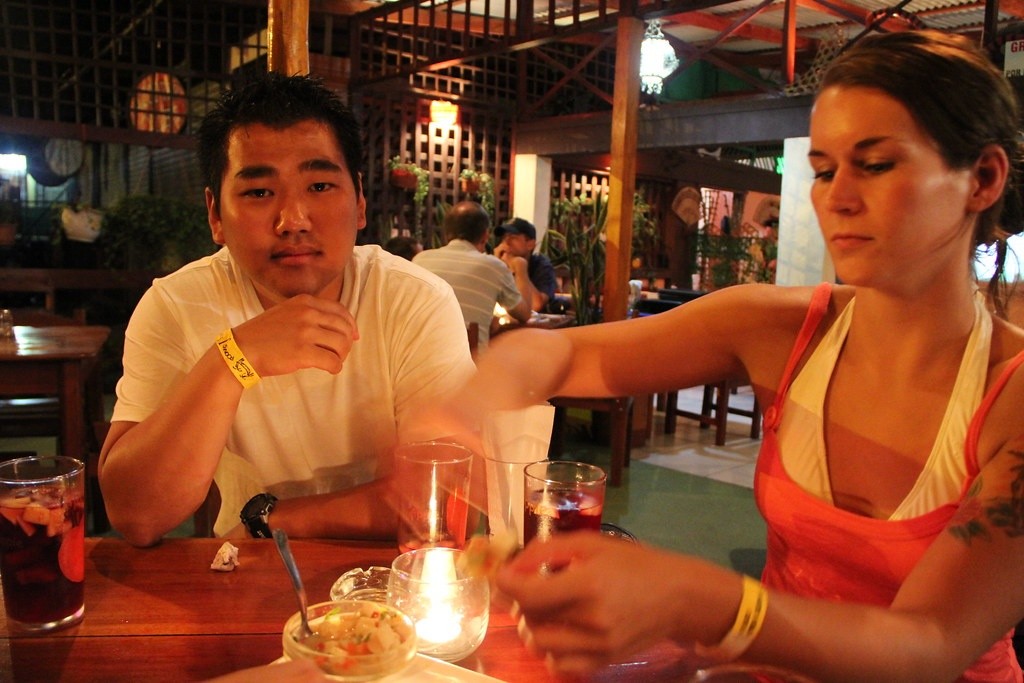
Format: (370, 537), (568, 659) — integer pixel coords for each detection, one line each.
(214, 328), (262, 390)
(692, 571), (769, 664)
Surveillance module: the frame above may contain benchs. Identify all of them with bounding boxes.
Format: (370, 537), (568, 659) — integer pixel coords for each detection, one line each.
(546, 289), (762, 487)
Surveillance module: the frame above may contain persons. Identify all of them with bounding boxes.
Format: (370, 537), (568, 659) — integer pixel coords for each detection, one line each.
(384, 197), (557, 355)
(484, 26), (1024, 683)
(96, 70), (486, 550)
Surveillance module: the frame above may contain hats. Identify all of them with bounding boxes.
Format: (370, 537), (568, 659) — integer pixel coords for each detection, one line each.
(494, 217), (536, 240)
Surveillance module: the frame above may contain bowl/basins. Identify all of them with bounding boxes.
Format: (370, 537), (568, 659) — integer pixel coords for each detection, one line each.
(281, 598), (420, 681)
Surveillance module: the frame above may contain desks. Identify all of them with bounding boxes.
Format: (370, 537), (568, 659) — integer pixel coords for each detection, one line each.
(0, 326), (111, 460)
(499, 315), (576, 333)
(0, 537), (736, 683)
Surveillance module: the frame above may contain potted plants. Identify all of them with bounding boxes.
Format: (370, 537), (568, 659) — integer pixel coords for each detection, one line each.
(539, 192), (657, 448)
(458, 169), (495, 247)
(52, 203), (108, 243)
(389, 156), (429, 240)
(104, 194), (217, 272)
(0, 200), (21, 245)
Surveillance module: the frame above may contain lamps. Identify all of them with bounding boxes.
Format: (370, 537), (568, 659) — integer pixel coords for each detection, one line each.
(638, 20), (679, 94)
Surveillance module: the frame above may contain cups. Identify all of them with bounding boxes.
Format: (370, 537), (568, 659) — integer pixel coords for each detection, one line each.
(0, 457), (85, 630)
(524, 460), (606, 574)
(395, 440), (473, 554)
(386, 547), (489, 664)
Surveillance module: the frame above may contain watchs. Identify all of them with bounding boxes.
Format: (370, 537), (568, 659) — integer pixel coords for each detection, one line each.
(239, 492), (279, 538)
